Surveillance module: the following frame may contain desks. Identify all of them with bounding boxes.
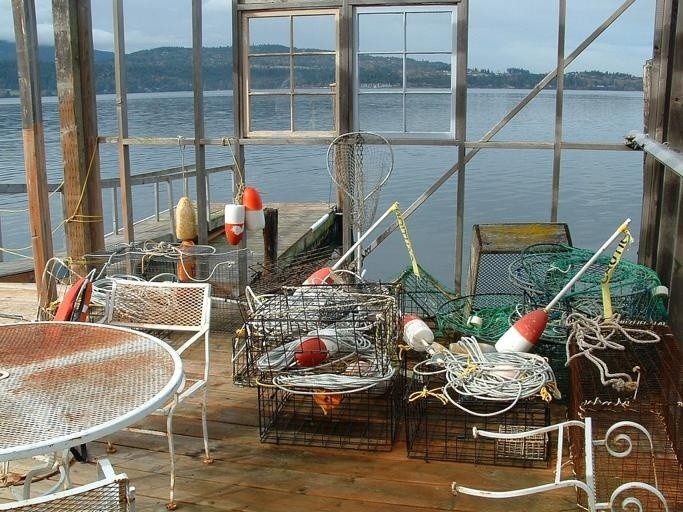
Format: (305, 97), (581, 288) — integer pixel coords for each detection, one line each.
(0, 321), (185, 500)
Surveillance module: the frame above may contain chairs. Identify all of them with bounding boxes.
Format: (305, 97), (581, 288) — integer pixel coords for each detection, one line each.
(451, 418), (668, 512)
(0, 459), (135, 512)
(48, 280), (213, 509)
